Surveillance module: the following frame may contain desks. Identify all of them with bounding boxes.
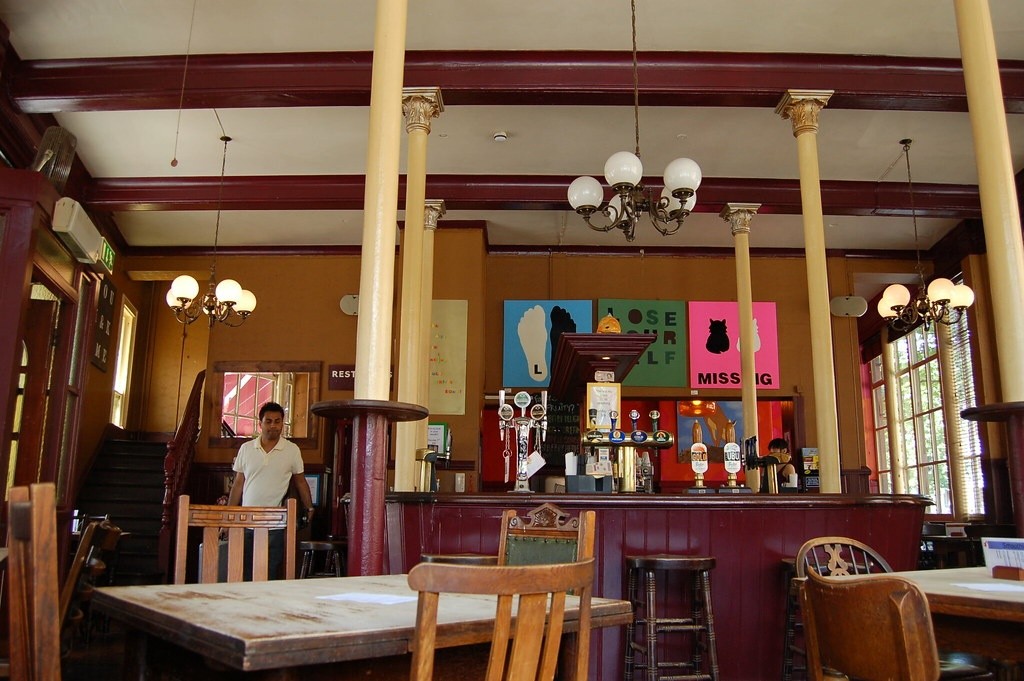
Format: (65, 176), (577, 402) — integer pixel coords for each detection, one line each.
(829, 567), (1024, 681)
(91, 572), (633, 681)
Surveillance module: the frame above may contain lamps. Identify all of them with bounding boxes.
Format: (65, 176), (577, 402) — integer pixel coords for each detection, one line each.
(877, 138), (975, 327)
(165, 135), (256, 329)
(567, 0), (703, 242)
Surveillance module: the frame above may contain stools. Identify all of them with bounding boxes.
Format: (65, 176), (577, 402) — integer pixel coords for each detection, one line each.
(620, 552), (720, 681)
(299, 537), (347, 578)
(781, 554), (873, 681)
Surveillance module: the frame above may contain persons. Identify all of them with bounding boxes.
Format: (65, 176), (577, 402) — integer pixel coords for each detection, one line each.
(217, 402), (313, 581)
(762, 438), (795, 490)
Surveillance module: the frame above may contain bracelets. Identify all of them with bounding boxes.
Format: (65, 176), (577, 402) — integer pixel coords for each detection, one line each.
(307, 507), (314, 512)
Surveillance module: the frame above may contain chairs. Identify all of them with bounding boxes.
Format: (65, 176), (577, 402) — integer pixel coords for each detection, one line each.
(498, 502), (595, 596)
(796, 537), (895, 578)
(799, 565), (993, 681)
(408, 557), (597, 681)
(0, 483), (301, 681)
